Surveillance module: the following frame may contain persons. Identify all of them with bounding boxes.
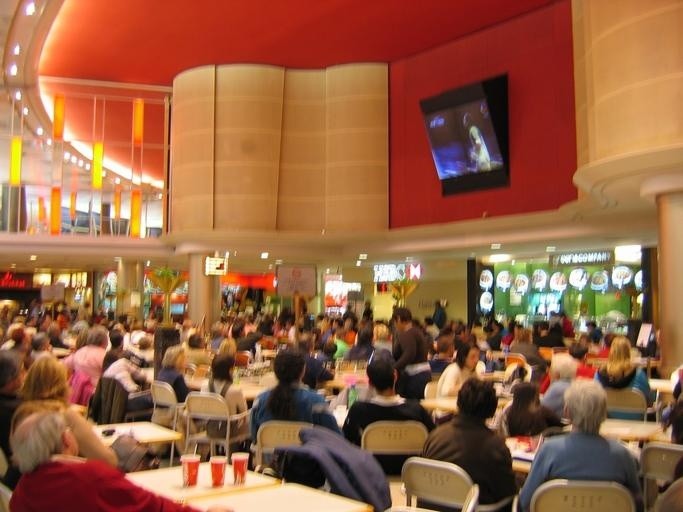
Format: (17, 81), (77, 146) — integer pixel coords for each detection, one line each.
(461, 112), (491, 173)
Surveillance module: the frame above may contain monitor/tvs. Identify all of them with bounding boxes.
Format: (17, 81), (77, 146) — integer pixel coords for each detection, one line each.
(420, 73), (509, 196)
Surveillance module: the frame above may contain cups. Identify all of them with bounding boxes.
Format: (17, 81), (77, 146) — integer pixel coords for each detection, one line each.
(231, 452), (250, 485)
(324, 358), (372, 373)
(231, 366), (275, 383)
(180, 453), (201, 487)
(209, 456), (227, 489)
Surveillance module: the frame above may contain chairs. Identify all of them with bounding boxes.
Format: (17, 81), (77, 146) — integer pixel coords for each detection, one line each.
(150, 373), (683, 512)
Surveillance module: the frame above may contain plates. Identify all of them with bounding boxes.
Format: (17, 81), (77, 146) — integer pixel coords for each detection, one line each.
(479, 265), (643, 292)
(480, 291), (494, 312)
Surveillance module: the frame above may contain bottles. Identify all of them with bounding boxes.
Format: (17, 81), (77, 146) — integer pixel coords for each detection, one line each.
(348, 382), (358, 413)
